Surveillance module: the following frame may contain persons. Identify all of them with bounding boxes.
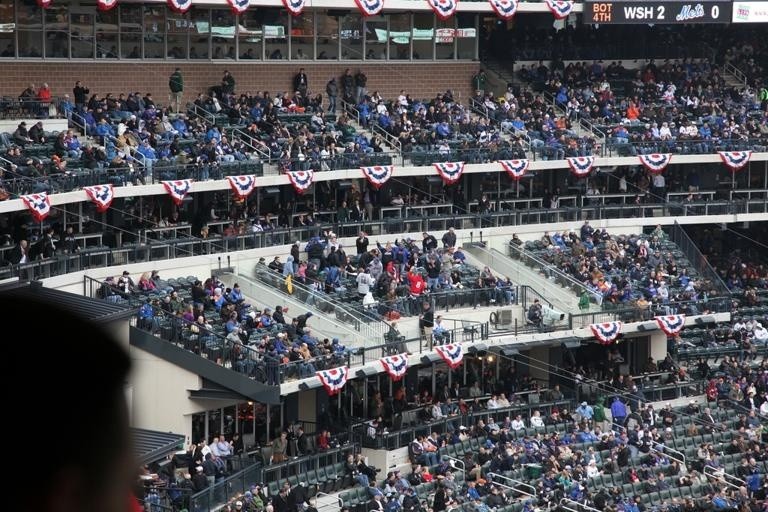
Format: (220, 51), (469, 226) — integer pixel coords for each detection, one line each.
(2, 2), (768, 511)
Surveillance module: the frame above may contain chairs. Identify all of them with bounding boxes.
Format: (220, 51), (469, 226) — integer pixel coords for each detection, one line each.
(1, 74), (768, 512)
